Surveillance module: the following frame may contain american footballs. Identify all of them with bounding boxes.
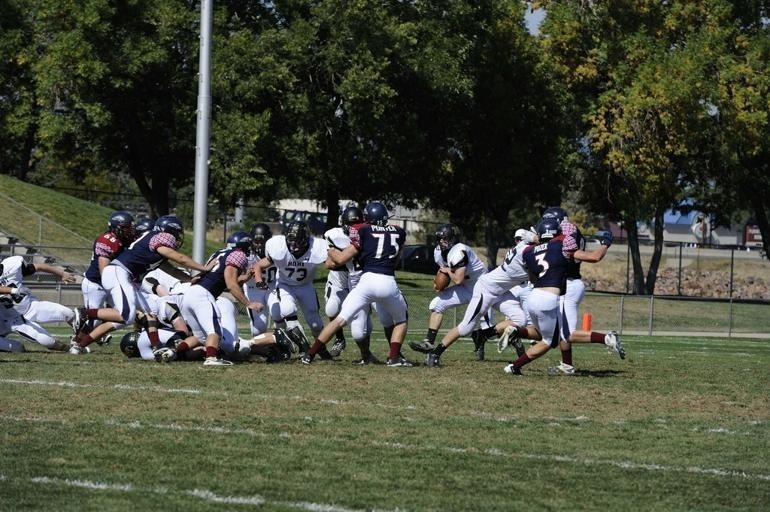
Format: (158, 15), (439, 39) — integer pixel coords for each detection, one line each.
(436, 273), (450, 290)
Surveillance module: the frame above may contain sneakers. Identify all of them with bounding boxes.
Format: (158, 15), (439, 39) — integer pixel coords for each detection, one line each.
(424, 351), (439, 367)
(74, 307), (88, 335)
(474, 335), (485, 360)
(317, 345), (331, 359)
(69, 344), (86, 355)
(330, 339), (345, 356)
(503, 363), (522, 375)
(498, 326), (518, 353)
(204, 357), (234, 366)
(513, 338), (525, 357)
(273, 328), (296, 354)
(605, 331), (625, 359)
(408, 341), (434, 353)
(96, 334), (112, 345)
(387, 356), (413, 367)
(548, 363), (576, 376)
(162, 349), (177, 362)
(301, 354), (313, 364)
(70, 337), (90, 353)
(292, 326), (311, 352)
(351, 358), (369, 365)
(151, 343), (171, 355)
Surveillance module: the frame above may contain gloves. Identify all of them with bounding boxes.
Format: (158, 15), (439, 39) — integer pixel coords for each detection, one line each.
(256, 281), (270, 290)
(599, 232), (614, 248)
(15, 287), (32, 297)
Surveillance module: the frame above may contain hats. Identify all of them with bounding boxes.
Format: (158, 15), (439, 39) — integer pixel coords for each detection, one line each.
(515, 229), (527, 237)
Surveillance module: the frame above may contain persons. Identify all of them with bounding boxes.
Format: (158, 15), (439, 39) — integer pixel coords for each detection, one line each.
(1, 193), (626, 379)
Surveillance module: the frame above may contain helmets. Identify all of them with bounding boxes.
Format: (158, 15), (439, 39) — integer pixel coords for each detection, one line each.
(249, 223), (272, 255)
(227, 232), (252, 256)
(436, 223), (461, 250)
(108, 212), (134, 242)
(286, 221), (310, 254)
(156, 216), (184, 249)
(120, 332), (141, 358)
(543, 207), (567, 223)
(134, 219), (154, 239)
(342, 207), (363, 235)
(363, 203), (388, 226)
(537, 219), (561, 242)
